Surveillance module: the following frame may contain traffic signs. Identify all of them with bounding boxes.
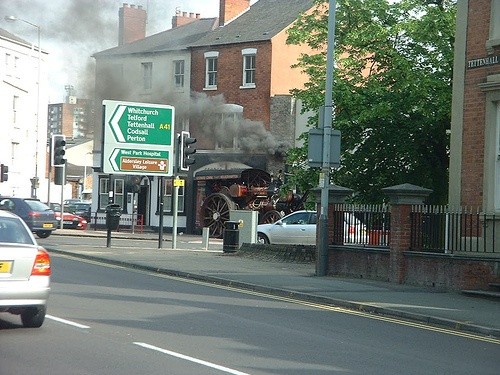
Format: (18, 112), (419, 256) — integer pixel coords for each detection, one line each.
(98, 101), (176, 179)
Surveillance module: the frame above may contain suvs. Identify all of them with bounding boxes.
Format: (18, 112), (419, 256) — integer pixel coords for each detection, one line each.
(0, 194), (58, 239)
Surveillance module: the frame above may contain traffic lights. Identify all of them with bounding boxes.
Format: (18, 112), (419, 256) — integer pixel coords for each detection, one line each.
(180, 131), (197, 171)
(51, 135), (66, 167)
(0, 163), (8, 182)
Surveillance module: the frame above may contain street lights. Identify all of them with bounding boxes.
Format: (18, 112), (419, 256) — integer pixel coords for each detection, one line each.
(4, 14), (41, 198)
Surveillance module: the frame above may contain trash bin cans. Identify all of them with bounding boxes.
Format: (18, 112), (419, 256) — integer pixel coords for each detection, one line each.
(106, 204), (121, 231)
(223, 221), (240, 253)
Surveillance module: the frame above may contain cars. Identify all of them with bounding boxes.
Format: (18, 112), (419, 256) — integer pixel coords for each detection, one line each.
(42, 199), (92, 230)
(256, 209), (369, 245)
(0, 210), (52, 329)
